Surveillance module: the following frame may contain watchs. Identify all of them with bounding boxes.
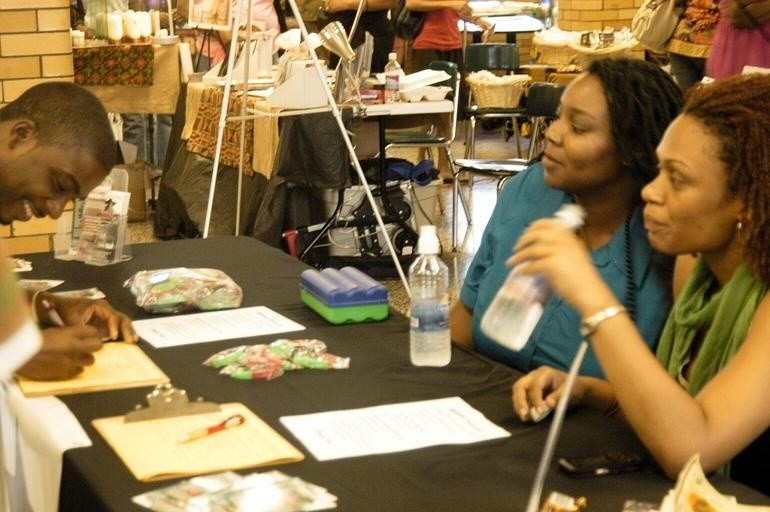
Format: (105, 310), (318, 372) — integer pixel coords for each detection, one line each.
(581, 307), (631, 336)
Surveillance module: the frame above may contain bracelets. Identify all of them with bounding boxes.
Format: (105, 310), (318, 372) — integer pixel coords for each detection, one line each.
(29, 289), (45, 325)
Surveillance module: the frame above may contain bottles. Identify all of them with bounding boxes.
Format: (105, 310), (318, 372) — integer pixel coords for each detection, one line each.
(384, 52), (400, 104)
(479, 202), (587, 352)
(408, 225), (452, 368)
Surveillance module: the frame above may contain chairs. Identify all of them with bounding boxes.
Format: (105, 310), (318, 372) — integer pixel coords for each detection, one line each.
(373, 42), (569, 223)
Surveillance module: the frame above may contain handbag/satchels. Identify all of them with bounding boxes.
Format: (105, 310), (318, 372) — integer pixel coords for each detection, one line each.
(728, 0), (770, 29)
(391, 0), (425, 40)
(632, 1), (685, 49)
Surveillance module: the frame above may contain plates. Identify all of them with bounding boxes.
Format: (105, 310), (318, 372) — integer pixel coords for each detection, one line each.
(152, 35), (179, 45)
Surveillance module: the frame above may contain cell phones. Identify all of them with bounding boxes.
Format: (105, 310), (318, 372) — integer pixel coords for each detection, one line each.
(558, 448), (642, 477)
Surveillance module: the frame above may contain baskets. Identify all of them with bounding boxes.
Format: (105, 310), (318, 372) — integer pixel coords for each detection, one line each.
(465, 76), (532, 108)
(533, 37), (629, 70)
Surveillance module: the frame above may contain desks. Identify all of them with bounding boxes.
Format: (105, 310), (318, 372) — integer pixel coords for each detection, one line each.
(159, 70), (455, 262)
(74, 39), (180, 209)
(0, 235), (768, 512)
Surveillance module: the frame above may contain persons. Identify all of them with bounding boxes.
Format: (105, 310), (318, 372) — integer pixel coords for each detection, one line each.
(0, 82), (139, 382)
(448, 56), (684, 374)
(318, 0), (496, 178)
(669, 1), (770, 93)
(506, 72), (770, 483)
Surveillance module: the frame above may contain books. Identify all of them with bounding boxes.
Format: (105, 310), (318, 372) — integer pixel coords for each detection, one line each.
(73, 189), (132, 264)
(91, 402), (305, 481)
(18, 341), (169, 402)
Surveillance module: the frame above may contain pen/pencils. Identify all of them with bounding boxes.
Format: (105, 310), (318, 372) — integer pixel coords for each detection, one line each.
(41, 300), (67, 327)
(176, 413), (245, 445)
